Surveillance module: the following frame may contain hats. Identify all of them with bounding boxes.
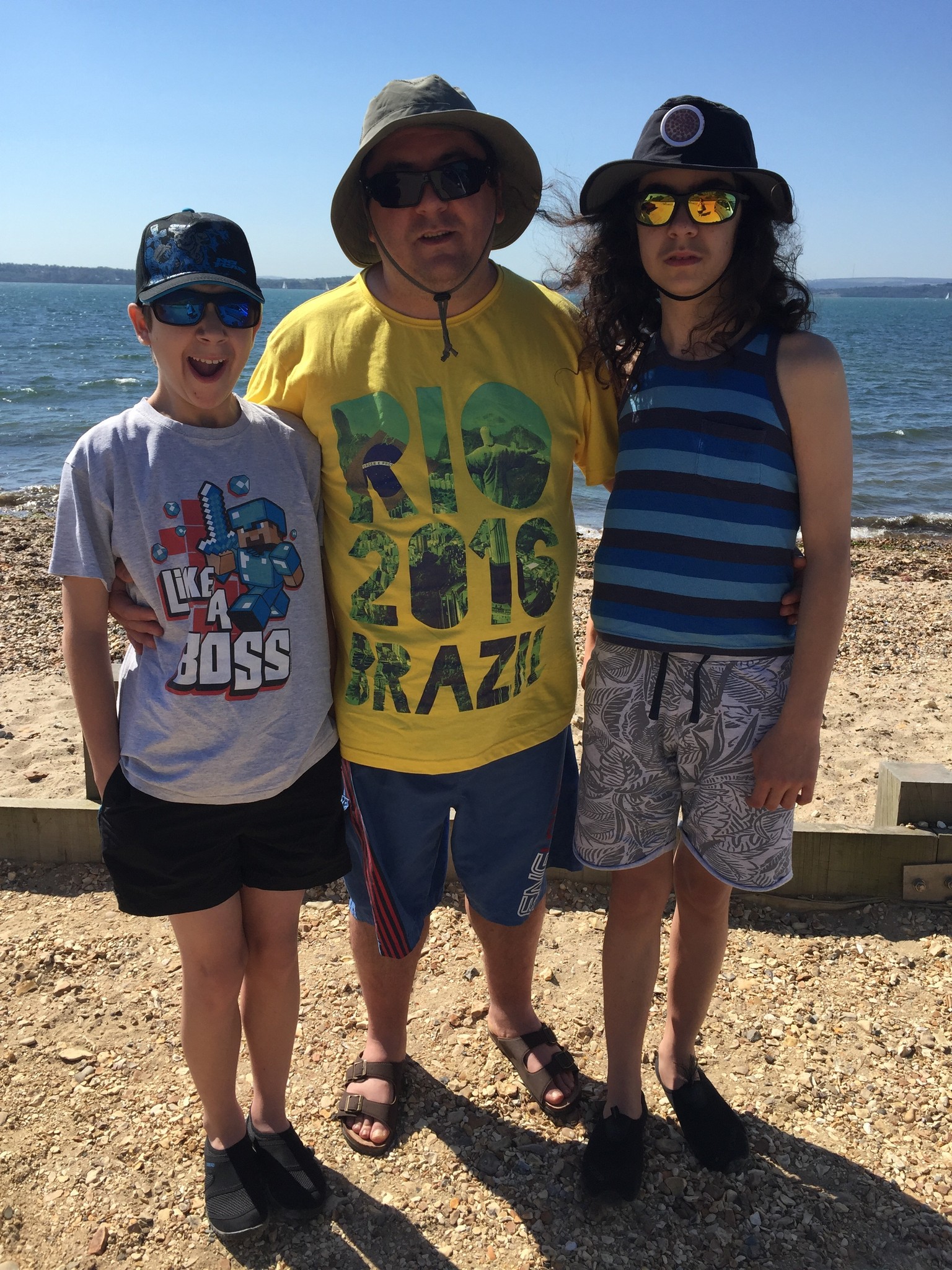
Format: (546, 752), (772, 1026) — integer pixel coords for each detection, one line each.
(137, 209), (265, 306)
(579, 94), (795, 227)
(330, 75), (542, 268)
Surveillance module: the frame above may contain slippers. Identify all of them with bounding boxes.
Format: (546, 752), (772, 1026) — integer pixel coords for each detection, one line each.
(487, 1018), (580, 1116)
(338, 1044), (407, 1155)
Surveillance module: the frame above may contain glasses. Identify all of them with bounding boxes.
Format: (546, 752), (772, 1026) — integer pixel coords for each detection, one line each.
(143, 286), (261, 329)
(362, 156), (500, 208)
(633, 189), (749, 227)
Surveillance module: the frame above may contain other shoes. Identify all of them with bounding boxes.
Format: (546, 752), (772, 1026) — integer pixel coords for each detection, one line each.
(203, 1127), (270, 1237)
(247, 1110), (326, 1218)
(656, 1052), (749, 1173)
(582, 1089), (649, 1209)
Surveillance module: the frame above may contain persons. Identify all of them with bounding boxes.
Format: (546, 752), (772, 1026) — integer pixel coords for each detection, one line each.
(105, 75), (811, 1156)
(53, 206), (338, 1240)
(523, 92), (858, 1201)
